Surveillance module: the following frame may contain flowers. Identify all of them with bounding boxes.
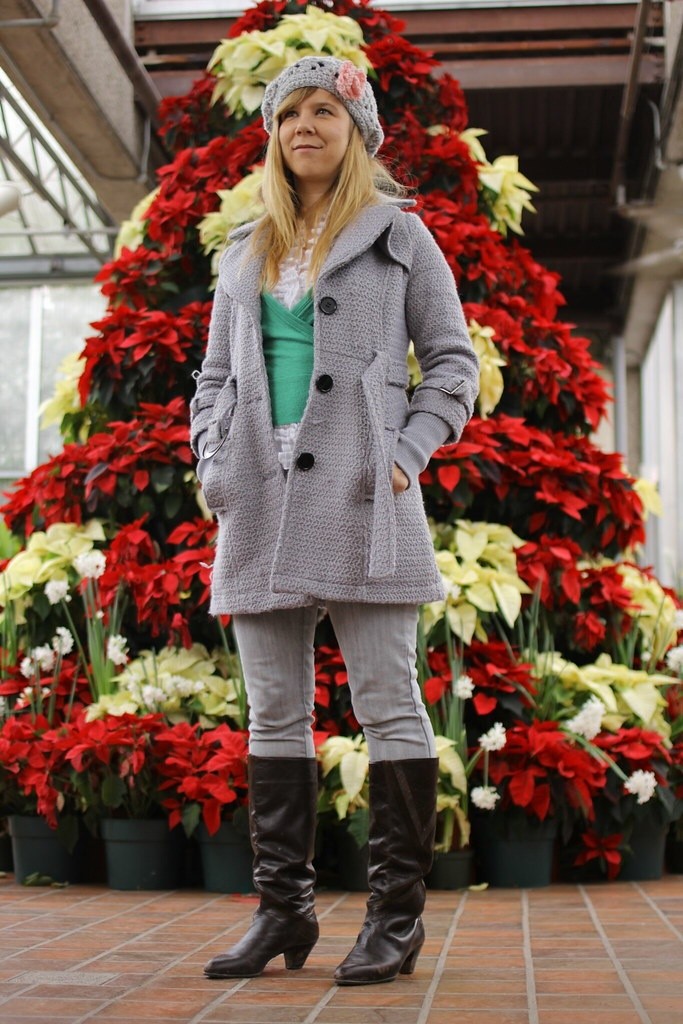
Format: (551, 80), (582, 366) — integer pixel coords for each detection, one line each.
(0, 0), (683, 886)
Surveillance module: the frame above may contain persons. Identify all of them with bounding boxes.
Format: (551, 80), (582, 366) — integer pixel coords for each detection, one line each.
(189, 57), (478, 984)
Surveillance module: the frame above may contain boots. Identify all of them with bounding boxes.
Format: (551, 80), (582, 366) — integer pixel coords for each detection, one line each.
(334, 757), (438, 985)
(202, 754), (319, 978)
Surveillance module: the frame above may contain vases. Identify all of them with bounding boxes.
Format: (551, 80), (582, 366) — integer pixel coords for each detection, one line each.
(343, 817), (373, 891)
(185, 822), (252, 892)
(89, 817), (175, 887)
(479, 808), (558, 889)
(568, 803), (671, 883)
(8, 813), (83, 886)
(423, 810), (474, 889)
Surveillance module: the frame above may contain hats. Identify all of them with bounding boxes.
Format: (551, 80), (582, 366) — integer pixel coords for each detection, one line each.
(261, 54), (383, 158)
